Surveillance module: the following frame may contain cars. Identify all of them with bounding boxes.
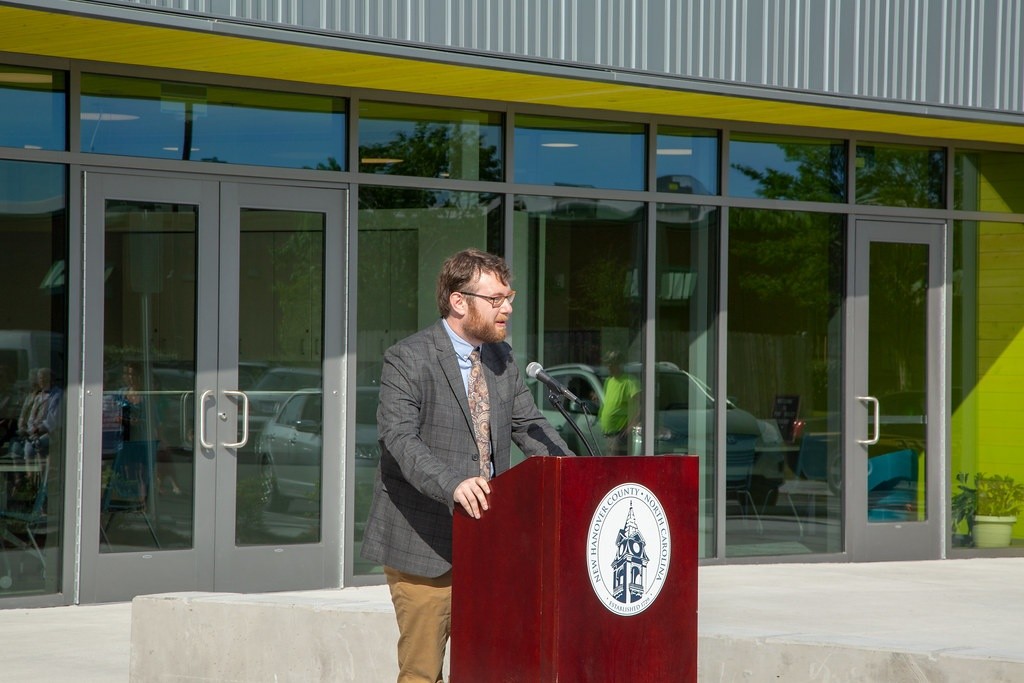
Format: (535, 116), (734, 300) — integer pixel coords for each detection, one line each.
(137, 348), (389, 465)
(253, 385), (380, 527)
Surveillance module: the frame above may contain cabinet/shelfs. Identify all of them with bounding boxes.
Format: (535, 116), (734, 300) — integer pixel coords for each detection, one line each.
(122, 229), (417, 362)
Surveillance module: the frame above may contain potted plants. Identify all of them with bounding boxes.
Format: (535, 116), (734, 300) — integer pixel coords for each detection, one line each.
(951, 471), (1024, 548)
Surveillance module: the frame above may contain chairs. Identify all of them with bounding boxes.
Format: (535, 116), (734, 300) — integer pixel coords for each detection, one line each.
(0, 456), (50, 590)
(735, 447), (803, 539)
(100, 438), (161, 554)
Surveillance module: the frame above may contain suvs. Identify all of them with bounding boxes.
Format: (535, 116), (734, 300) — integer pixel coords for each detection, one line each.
(524, 359), (789, 511)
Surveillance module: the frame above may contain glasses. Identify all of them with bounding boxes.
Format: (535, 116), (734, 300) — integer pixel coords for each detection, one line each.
(451, 291), (516, 308)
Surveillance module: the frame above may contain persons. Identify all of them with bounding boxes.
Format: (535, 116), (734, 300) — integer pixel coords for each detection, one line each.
(12, 362), (163, 521)
(597, 351), (642, 456)
(359, 248), (577, 683)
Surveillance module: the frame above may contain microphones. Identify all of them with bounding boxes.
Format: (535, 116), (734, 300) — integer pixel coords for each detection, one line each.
(526, 362), (583, 406)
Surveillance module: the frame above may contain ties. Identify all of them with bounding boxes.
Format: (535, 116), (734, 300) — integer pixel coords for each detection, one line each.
(468, 351), (491, 485)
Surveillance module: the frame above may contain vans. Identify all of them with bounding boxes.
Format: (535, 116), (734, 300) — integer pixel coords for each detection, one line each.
(1, 330), (68, 400)
